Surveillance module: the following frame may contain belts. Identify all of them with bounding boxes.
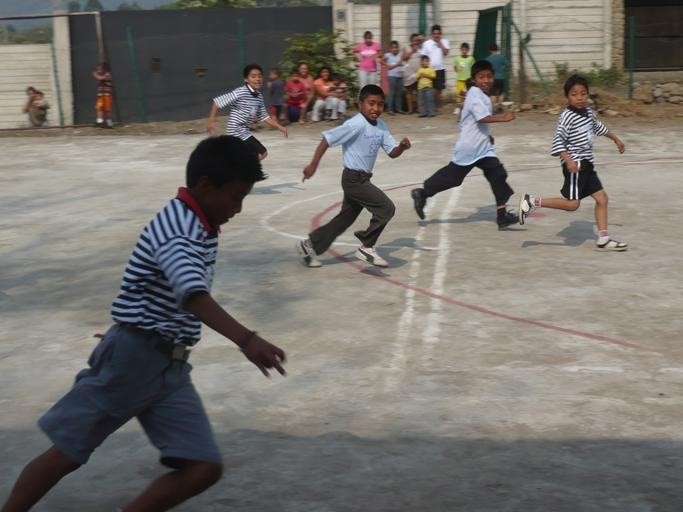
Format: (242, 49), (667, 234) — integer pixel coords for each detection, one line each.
(120, 323), (189, 360)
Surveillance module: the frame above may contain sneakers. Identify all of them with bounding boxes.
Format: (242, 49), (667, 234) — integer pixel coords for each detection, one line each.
(520, 194), (534, 225)
(354, 247), (389, 266)
(412, 189), (426, 219)
(597, 237), (628, 250)
(497, 213), (519, 227)
(301, 239), (321, 266)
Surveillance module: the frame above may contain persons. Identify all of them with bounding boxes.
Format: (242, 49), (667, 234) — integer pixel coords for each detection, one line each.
(0, 134), (287, 511)
(517, 73), (630, 253)
(31, 91), (43, 110)
(204, 62), (289, 162)
(264, 22), (514, 127)
(20, 86), (49, 128)
(299, 83), (413, 269)
(409, 60), (526, 230)
(92, 62), (113, 128)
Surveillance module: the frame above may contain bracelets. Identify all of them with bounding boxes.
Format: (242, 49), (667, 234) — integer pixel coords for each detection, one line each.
(239, 330), (256, 353)
